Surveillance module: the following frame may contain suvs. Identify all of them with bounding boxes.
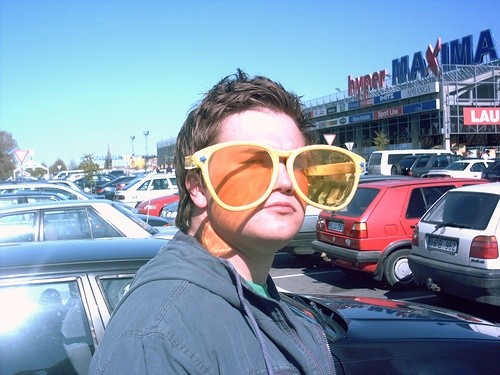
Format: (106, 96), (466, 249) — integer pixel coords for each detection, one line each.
(390, 153), (468, 179)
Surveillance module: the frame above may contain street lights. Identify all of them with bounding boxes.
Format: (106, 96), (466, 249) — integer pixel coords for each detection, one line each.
(145, 130), (149, 164)
(129, 134), (136, 157)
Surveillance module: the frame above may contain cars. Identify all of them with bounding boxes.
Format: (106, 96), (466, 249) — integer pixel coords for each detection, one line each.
(427, 157), (500, 183)
(406, 183), (500, 311)
(311, 173), (495, 291)
(0, 165), (340, 271)
(0, 233), (500, 375)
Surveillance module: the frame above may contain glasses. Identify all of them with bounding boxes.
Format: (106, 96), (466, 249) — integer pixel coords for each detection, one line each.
(184, 141), (366, 210)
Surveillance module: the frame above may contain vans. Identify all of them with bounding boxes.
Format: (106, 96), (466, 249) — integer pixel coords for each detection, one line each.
(366, 148), (454, 177)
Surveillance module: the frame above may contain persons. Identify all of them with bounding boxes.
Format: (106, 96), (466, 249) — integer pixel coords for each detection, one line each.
(453, 142), (496, 159)
(88, 66), (366, 375)
